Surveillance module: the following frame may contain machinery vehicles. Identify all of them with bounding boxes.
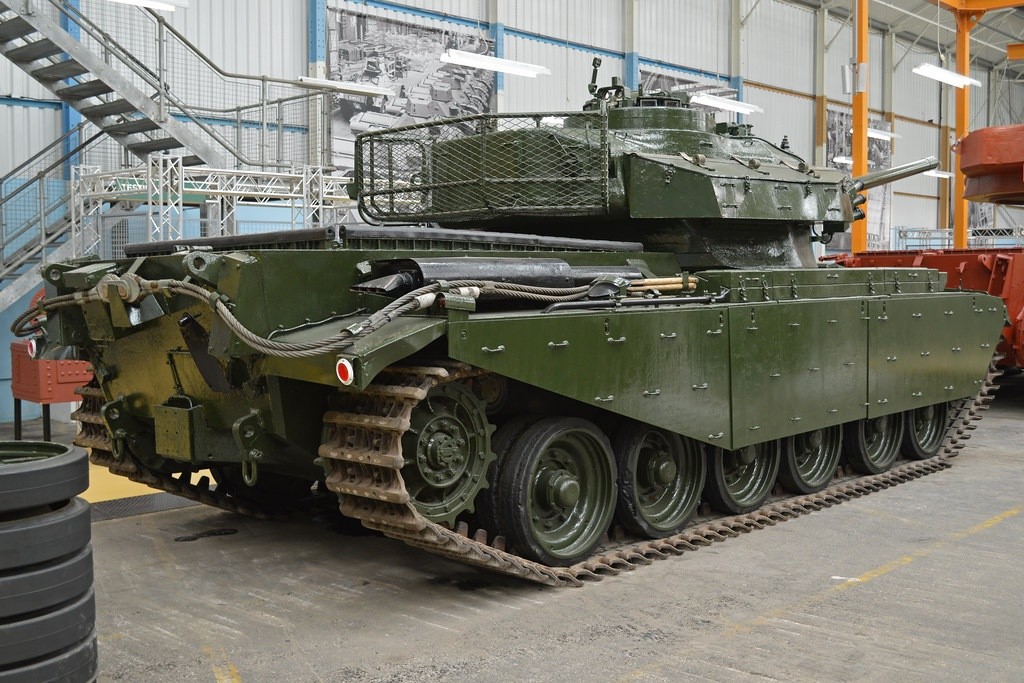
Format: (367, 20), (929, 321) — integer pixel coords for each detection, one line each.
(8, 56), (1015, 590)
(336, 27), (497, 146)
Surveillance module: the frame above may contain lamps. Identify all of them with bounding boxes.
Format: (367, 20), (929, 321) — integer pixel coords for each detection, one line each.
(913, 1), (981, 88)
(848, 18), (901, 141)
(688, 0), (758, 116)
(441, 0), (550, 78)
